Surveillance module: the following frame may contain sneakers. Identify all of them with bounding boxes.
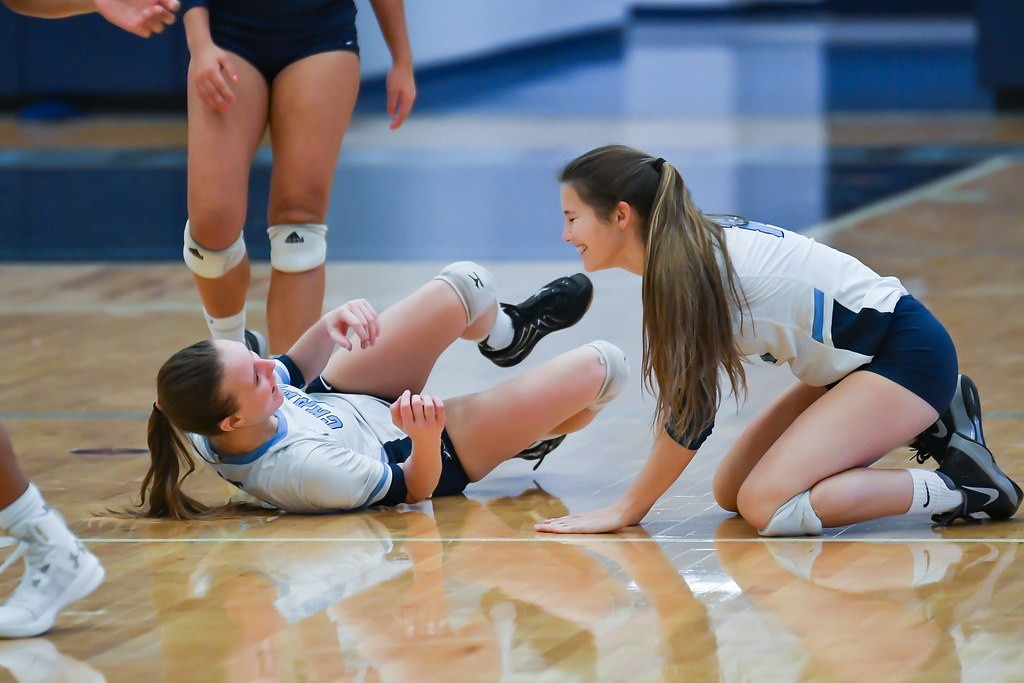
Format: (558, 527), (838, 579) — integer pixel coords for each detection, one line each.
(477, 273), (594, 367)
(931, 432), (1024, 524)
(0, 507), (106, 638)
(909, 373), (986, 464)
(245, 330), (267, 359)
(512, 434), (566, 471)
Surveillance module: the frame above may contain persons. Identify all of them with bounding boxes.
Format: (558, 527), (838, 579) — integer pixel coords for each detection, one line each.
(0, 425), (104, 638)
(182, 0), (416, 357)
(130, 259), (626, 515)
(5, 1), (179, 39)
(187, 505), (628, 683)
(534, 142), (1022, 537)
(0, 640), (104, 683)
(537, 514), (1023, 682)
